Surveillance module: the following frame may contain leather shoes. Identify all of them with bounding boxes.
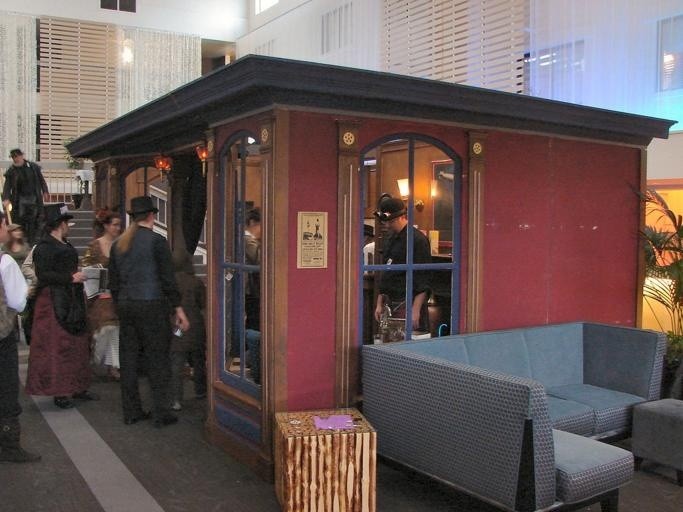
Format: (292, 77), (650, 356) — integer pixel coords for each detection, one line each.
(124, 409), (152, 424)
(71, 390), (101, 402)
(54, 395), (74, 409)
(153, 414), (179, 427)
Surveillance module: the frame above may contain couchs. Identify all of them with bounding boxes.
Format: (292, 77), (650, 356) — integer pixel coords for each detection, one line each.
(362, 321), (665, 511)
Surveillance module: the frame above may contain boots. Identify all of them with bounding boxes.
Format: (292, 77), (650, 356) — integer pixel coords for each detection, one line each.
(0, 417), (43, 463)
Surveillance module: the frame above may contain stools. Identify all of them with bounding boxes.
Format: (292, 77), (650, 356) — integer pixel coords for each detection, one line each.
(631, 398), (683, 484)
(277, 409), (376, 511)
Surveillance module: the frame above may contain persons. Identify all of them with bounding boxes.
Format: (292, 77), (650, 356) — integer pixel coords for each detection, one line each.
(25, 212), (100, 409)
(0, 211), (42, 463)
(373, 196), (433, 330)
(2, 148), (50, 245)
(82, 210), (124, 381)
(245, 208), (262, 331)
(172, 248), (209, 410)
(108, 196), (179, 428)
(3, 219), (31, 266)
(21, 243), (39, 288)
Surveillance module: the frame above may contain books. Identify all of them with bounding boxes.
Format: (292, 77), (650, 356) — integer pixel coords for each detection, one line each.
(403, 328), (431, 342)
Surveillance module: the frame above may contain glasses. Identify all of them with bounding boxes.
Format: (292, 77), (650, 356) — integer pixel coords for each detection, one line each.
(376, 212), (390, 217)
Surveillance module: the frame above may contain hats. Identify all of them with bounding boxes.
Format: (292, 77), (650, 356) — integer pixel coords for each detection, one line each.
(380, 198), (407, 220)
(364, 224), (375, 237)
(6, 223), (22, 232)
(44, 202), (74, 225)
(127, 195), (160, 215)
(8, 149), (24, 157)
